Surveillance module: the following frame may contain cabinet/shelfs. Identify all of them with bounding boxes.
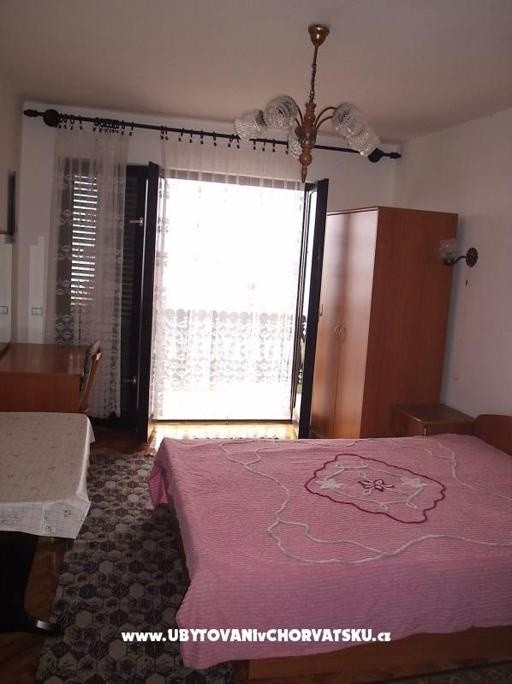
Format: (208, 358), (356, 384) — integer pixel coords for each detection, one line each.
(307, 205), (460, 444)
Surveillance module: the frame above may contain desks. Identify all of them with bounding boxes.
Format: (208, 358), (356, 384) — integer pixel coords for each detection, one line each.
(1, 339), (95, 683)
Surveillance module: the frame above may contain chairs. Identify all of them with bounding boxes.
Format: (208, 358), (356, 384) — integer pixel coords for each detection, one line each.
(77, 338), (104, 412)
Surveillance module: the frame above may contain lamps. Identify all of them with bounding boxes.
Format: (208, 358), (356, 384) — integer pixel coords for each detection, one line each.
(234, 24), (383, 186)
(437, 238), (479, 268)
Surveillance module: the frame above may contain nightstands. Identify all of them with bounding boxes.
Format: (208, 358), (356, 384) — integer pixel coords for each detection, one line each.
(388, 399), (472, 439)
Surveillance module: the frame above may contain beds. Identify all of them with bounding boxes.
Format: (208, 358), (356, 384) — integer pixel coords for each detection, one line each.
(154, 414), (511, 682)
